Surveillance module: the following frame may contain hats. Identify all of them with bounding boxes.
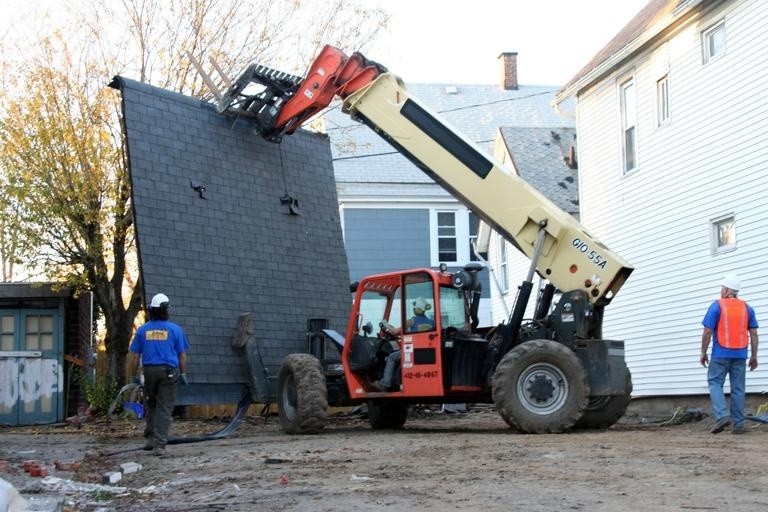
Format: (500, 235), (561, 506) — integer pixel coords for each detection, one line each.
(411, 296), (428, 311)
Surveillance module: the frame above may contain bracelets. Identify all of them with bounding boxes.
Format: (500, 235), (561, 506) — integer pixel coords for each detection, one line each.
(701, 352), (706, 355)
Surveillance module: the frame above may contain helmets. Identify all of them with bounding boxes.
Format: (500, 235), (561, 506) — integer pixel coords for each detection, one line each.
(149, 293), (169, 307)
(720, 273), (742, 292)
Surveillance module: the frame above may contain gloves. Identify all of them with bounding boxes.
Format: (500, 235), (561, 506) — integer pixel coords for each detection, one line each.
(179, 374), (188, 385)
(131, 376), (138, 384)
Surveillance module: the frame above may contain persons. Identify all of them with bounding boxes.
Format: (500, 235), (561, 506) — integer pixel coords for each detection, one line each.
(700, 275), (758, 434)
(129, 292), (190, 456)
(374, 297), (434, 392)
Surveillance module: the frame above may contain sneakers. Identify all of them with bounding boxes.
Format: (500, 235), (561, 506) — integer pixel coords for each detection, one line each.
(372, 380), (389, 391)
(710, 418), (743, 434)
(143, 436), (164, 455)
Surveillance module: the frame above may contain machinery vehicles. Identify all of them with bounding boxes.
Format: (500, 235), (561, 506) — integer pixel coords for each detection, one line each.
(184, 43), (635, 434)
